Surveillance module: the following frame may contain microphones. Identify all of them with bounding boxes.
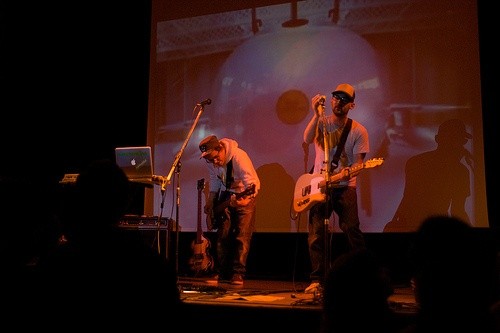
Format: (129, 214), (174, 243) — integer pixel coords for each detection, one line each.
(196, 98), (212, 108)
(319, 95), (326, 105)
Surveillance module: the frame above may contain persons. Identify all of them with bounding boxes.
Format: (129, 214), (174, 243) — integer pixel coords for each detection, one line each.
(199, 136), (261, 284)
(303, 84), (369, 294)
(320, 217), (500, 333)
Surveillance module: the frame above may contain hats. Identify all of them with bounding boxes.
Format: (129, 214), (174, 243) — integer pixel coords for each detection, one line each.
(199, 135), (220, 159)
(331, 83), (355, 101)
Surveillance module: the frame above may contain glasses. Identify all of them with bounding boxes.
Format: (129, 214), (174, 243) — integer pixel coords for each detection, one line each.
(332, 94), (351, 103)
(206, 151), (219, 163)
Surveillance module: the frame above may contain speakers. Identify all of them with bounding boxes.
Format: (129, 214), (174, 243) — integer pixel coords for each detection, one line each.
(116, 228), (169, 289)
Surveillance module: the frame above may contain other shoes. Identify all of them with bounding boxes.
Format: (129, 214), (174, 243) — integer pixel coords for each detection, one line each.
(202, 276), (223, 286)
(305, 282), (322, 294)
(231, 274), (243, 285)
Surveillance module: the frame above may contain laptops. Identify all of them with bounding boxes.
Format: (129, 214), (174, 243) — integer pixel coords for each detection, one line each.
(116, 146), (166, 182)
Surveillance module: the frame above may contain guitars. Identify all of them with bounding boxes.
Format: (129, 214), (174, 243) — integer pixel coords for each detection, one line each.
(206, 183), (256, 231)
(186, 178), (214, 272)
(292, 156), (385, 213)
